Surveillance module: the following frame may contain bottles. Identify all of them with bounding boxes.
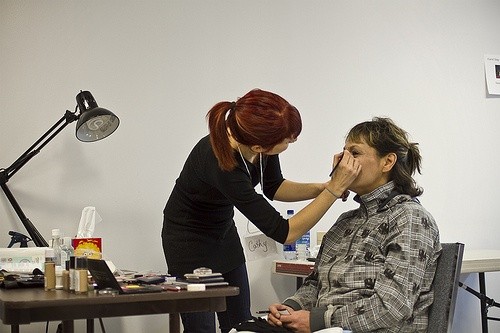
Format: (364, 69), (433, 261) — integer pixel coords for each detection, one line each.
(295, 229), (310, 261)
(44, 229), (88, 294)
(283, 210), (296, 261)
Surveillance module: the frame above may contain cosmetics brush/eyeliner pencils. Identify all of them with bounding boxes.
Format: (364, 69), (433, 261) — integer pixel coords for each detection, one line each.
(329, 153), (344, 177)
(256, 311), (271, 314)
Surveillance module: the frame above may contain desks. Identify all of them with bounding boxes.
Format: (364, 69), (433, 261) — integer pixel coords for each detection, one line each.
(0, 272), (240, 333)
(271, 249), (500, 333)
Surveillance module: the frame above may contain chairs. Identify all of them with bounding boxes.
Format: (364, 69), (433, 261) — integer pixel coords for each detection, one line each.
(425, 242), (465, 333)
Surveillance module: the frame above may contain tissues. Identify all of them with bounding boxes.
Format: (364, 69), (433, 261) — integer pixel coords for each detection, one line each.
(71, 206), (103, 259)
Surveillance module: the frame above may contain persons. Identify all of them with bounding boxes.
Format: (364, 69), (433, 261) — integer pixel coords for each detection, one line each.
(237, 117), (442, 333)
(162, 89), (362, 333)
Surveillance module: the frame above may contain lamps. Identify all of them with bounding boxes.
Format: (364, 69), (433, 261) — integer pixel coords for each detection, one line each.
(0, 90), (121, 247)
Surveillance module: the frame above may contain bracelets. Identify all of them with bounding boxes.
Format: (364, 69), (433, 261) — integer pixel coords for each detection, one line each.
(325, 187), (340, 199)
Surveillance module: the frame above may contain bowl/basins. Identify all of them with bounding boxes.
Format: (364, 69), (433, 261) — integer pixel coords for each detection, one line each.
(307, 245), (320, 259)
